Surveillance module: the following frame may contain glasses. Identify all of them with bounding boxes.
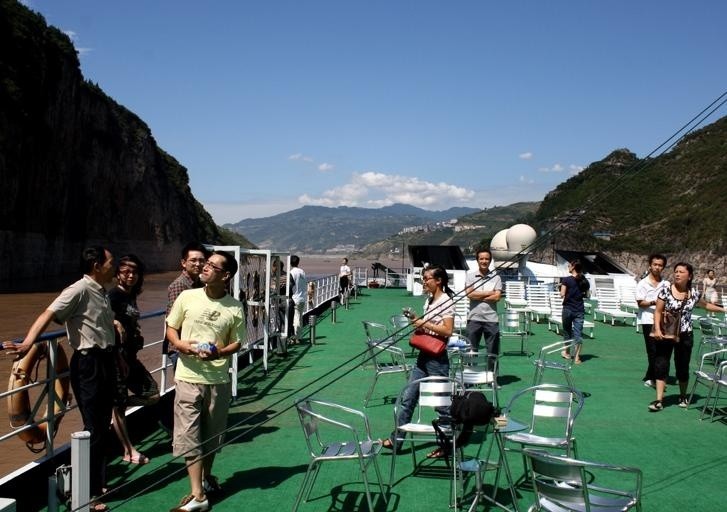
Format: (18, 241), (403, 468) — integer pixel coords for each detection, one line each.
(204, 261), (225, 271)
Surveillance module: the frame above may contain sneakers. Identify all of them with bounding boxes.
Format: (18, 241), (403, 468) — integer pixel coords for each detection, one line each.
(123, 453), (149, 464)
(561, 350), (571, 358)
(170, 475), (223, 512)
(648, 400), (663, 410)
(678, 396), (688, 408)
(382, 439), (400, 450)
(426, 449), (444, 458)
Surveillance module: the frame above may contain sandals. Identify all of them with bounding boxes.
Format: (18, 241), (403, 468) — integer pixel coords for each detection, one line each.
(89, 500), (108, 512)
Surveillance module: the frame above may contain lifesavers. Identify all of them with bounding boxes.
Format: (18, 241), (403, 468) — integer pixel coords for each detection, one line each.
(6, 338), (69, 443)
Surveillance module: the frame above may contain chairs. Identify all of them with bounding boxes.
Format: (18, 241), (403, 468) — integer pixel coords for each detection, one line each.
(523, 443), (641, 511)
(291, 396), (389, 511)
(529, 337), (583, 400)
(687, 315), (727, 426)
(363, 338), (417, 408)
(448, 331), (502, 410)
(361, 319), (397, 364)
(481, 381), (583, 498)
(405, 264), (727, 337)
(388, 314), (417, 356)
(387, 373), (467, 488)
(497, 311), (527, 356)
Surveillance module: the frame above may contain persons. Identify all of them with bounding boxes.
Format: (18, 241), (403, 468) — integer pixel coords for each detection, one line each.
(289, 255), (306, 344)
(649, 262), (727, 411)
(270, 259), (296, 345)
(107, 254), (158, 464)
(338, 258), (352, 311)
(559, 259), (591, 366)
(161, 245), (208, 371)
(465, 247), (503, 391)
(635, 255), (667, 392)
(379, 264), (456, 458)
(3, 245), (114, 510)
(702, 270), (718, 316)
(164, 250), (246, 512)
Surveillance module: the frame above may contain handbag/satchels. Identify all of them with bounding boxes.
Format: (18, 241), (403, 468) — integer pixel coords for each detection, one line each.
(649, 310), (681, 343)
(451, 391), (492, 424)
(409, 330), (446, 356)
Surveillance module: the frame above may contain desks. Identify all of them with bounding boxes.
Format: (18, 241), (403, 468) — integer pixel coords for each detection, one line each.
(430, 416), (528, 511)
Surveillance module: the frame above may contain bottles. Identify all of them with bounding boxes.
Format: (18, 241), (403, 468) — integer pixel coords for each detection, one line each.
(191, 341), (216, 353)
